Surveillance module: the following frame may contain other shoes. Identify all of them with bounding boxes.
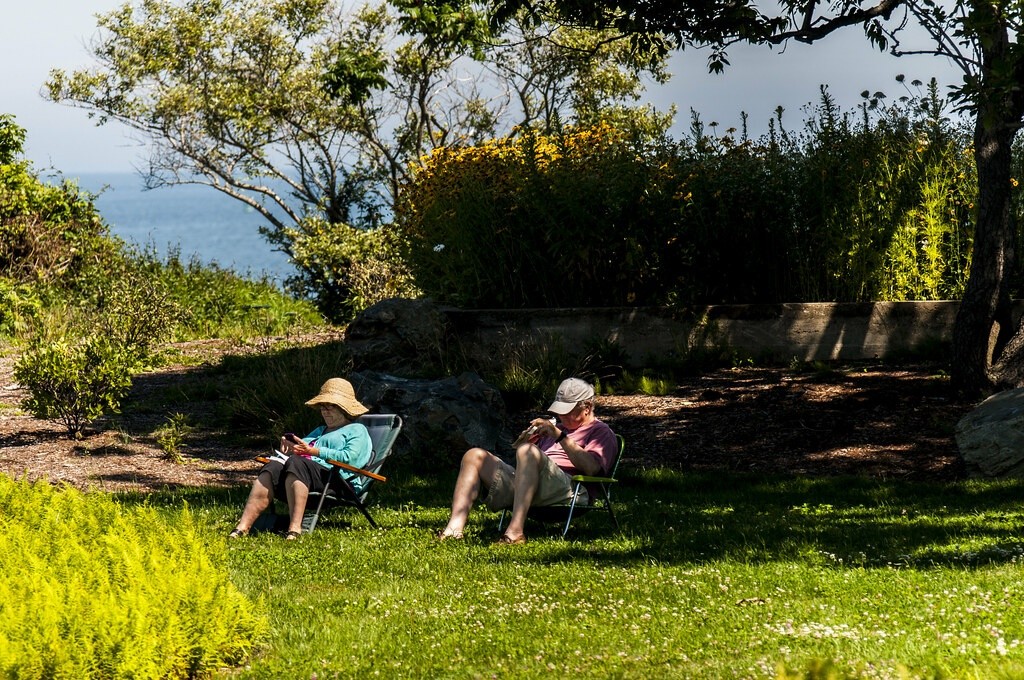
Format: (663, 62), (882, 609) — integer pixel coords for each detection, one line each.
(286, 531), (301, 541)
(498, 534), (526, 546)
(228, 529), (245, 540)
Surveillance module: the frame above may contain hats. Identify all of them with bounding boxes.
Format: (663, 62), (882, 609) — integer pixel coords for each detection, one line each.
(548, 377), (595, 415)
(304, 378), (369, 420)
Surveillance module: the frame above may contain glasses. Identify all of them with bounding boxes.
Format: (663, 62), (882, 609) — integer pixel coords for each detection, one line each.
(316, 404), (335, 410)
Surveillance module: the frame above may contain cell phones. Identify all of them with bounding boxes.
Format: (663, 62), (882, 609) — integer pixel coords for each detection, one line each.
(285, 433), (298, 444)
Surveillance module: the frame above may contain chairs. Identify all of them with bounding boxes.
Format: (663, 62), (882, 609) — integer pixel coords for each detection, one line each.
(498, 434), (625, 541)
(255, 414), (402, 534)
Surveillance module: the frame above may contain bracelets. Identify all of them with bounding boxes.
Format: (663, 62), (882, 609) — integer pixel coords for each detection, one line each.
(555, 431), (566, 444)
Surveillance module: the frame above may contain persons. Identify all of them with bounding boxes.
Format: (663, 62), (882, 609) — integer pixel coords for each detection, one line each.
(439, 377), (618, 543)
(228, 377), (373, 540)
